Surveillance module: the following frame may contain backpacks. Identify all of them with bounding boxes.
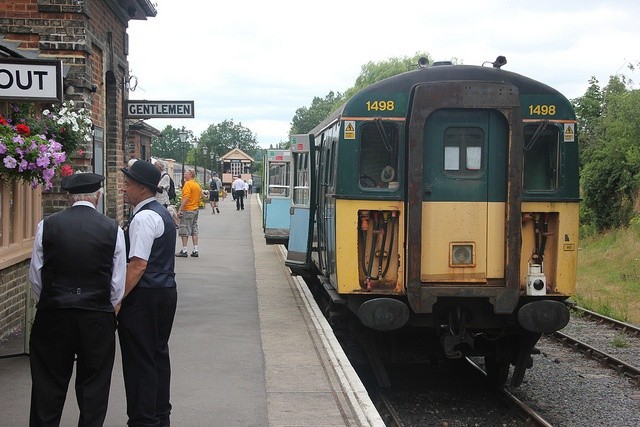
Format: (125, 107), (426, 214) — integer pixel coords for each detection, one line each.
(209, 177), (217, 190)
(160, 172), (177, 201)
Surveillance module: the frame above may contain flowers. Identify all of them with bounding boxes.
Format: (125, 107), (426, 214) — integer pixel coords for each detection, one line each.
(41, 98), (96, 160)
(0, 112), (73, 192)
(7, 101), (40, 134)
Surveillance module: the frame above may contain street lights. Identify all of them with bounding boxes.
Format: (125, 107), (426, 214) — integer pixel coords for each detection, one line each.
(215, 153), (220, 176)
(178, 125), (188, 188)
(209, 148), (216, 177)
(202, 142), (208, 187)
(192, 138), (200, 176)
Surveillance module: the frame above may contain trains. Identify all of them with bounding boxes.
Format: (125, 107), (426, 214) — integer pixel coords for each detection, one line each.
(260, 54), (583, 388)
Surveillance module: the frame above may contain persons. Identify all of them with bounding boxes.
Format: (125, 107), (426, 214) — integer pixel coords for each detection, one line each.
(248, 177), (253, 194)
(117, 159), (178, 427)
(177, 169), (202, 257)
(243, 180), (249, 199)
(29, 173), (126, 427)
(234, 174), (245, 210)
(232, 178), (237, 200)
(208, 172), (222, 213)
(154, 159), (171, 210)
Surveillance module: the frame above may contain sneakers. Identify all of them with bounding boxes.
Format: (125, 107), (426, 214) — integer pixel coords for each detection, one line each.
(190, 250), (199, 257)
(175, 250), (189, 257)
(216, 208), (219, 213)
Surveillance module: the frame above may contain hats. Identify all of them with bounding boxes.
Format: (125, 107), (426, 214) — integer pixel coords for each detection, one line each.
(60, 172), (106, 195)
(121, 160), (164, 193)
